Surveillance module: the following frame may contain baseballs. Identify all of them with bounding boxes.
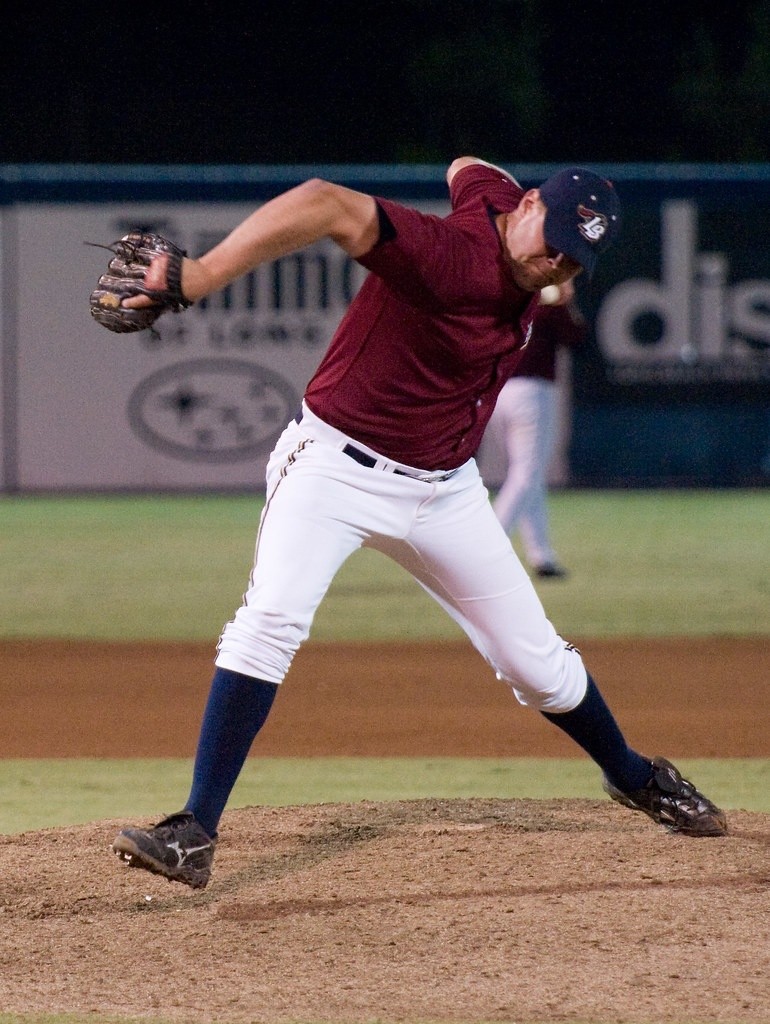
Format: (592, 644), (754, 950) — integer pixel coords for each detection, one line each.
(538, 281), (559, 305)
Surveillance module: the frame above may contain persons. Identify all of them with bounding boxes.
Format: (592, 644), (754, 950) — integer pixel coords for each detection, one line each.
(491, 281), (574, 578)
(91, 155), (725, 892)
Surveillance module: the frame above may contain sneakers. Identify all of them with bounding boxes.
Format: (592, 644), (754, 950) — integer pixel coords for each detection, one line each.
(112, 810), (219, 889)
(602, 749), (727, 837)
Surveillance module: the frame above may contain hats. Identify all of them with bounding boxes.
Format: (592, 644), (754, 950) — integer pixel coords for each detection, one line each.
(538, 167), (621, 282)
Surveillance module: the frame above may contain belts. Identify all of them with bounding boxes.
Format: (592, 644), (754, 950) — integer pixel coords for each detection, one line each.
(295, 407), (460, 483)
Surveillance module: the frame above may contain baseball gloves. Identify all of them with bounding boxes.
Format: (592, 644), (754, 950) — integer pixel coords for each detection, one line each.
(88, 232), (183, 334)
